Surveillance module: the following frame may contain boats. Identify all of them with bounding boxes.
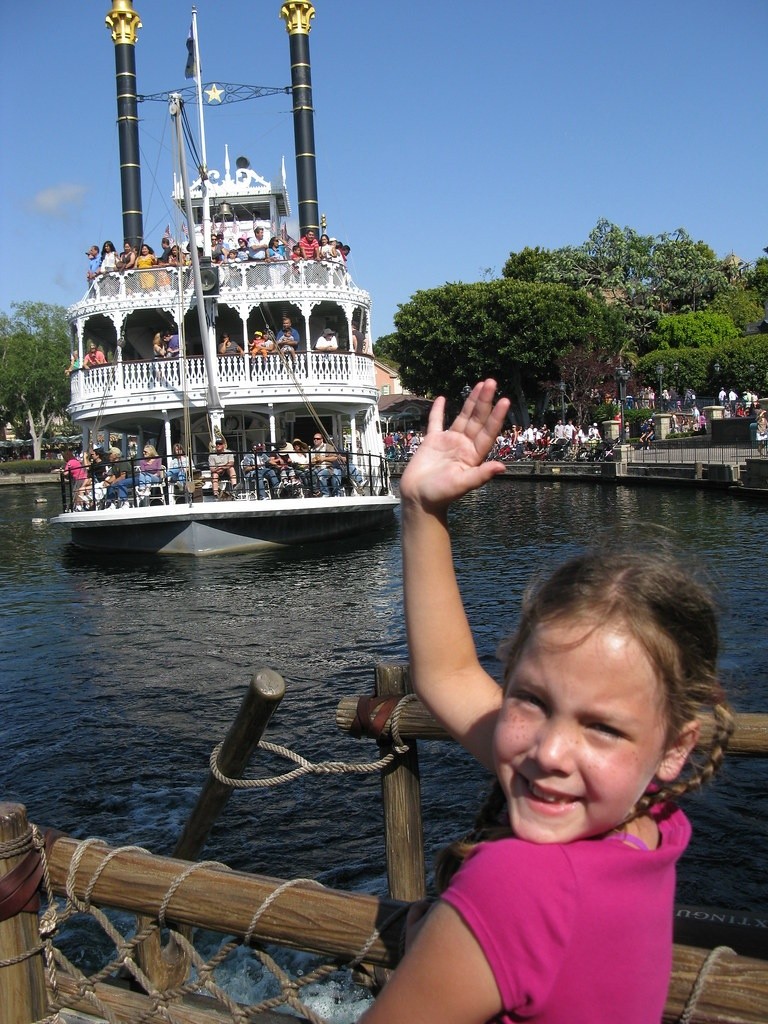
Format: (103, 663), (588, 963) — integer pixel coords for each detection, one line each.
(47, 1), (405, 557)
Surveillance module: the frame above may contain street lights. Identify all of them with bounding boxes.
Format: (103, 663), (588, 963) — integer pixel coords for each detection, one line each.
(613, 365), (632, 445)
(559, 380), (567, 425)
(657, 364), (666, 414)
(674, 362), (679, 411)
(715, 364), (720, 406)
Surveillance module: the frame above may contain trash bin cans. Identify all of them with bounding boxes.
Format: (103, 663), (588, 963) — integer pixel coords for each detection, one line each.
(750, 423), (761, 449)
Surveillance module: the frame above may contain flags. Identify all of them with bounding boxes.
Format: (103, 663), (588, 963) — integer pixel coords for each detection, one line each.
(164, 212), (287, 244)
(184, 17), (202, 80)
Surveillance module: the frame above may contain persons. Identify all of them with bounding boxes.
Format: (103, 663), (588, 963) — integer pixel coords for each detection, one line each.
(353, 326), (363, 352)
(152, 329), (179, 359)
(64, 343), (108, 376)
(248, 317), (300, 364)
(315, 328), (338, 352)
(383, 419), (601, 462)
(88, 226), (350, 299)
(593, 386), (759, 450)
(61, 433), (367, 511)
(218, 331), (244, 354)
(356, 378), (736, 1024)
(755, 409), (768, 457)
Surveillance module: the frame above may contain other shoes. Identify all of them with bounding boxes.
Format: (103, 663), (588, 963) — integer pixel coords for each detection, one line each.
(77, 494), (93, 504)
(104, 505), (115, 510)
(276, 482), (284, 494)
(119, 504), (129, 509)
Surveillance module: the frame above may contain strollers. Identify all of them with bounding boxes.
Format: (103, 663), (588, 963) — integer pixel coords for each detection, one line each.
(635, 430), (655, 450)
(386, 444), (419, 462)
(486, 437), (623, 462)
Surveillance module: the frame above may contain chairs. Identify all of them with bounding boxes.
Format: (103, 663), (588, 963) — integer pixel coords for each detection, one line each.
(118, 466), (167, 509)
(241, 460), (272, 500)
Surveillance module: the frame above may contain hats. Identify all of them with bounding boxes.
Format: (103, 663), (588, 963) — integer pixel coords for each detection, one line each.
(322, 328), (334, 337)
(329, 238), (336, 241)
(292, 438), (307, 449)
(217, 232), (224, 239)
(220, 332), (228, 337)
(254, 331), (262, 336)
(274, 440), (287, 448)
(215, 437), (224, 443)
(251, 441), (262, 451)
(85, 251), (90, 254)
(238, 237), (249, 244)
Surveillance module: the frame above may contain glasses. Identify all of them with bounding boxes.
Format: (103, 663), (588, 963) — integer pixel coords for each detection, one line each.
(274, 240), (279, 242)
(313, 438), (322, 440)
(211, 239), (217, 241)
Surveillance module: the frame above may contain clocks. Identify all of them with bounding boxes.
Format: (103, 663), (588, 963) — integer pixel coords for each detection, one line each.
(224, 416), (240, 430)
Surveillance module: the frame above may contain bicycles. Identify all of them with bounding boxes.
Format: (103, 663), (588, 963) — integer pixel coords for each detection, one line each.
(758, 428), (768, 458)
